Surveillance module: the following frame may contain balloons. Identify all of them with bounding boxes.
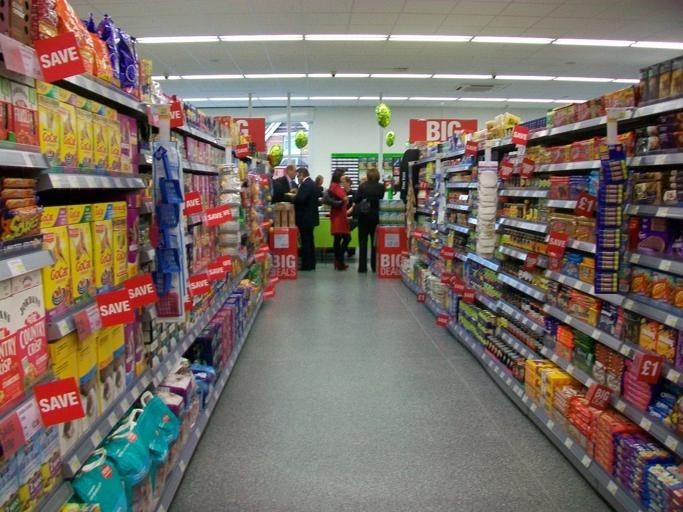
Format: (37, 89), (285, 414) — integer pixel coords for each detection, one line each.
(269, 145), (283, 166)
(386, 131), (394, 146)
(295, 130), (308, 148)
(376, 103), (391, 127)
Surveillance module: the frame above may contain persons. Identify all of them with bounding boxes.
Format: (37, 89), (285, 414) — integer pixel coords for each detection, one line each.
(277, 165), (298, 201)
(289, 168), (319, 271)
(269, 167), (284, 204)
(315, 176), (323, 206)
(329, 167), (352, 270)
(352, 168), (385, 272)
(343, 177), (359, 231)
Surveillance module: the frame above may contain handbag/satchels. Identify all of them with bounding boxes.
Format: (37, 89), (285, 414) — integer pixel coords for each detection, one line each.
(323, 189), (344, 208)
(356, 198), (371, 215)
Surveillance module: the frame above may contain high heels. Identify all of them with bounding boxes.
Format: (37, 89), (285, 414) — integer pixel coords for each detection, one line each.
(335, 259), (348, 271)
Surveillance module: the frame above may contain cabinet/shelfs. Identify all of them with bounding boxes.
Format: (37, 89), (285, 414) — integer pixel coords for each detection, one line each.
(0, 32), (273, 512)
(400, 99), (683, 509)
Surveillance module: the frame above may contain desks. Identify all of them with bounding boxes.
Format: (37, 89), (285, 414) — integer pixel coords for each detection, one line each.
(273, 228), (298, 279)
(375, 225), (408, 277)
